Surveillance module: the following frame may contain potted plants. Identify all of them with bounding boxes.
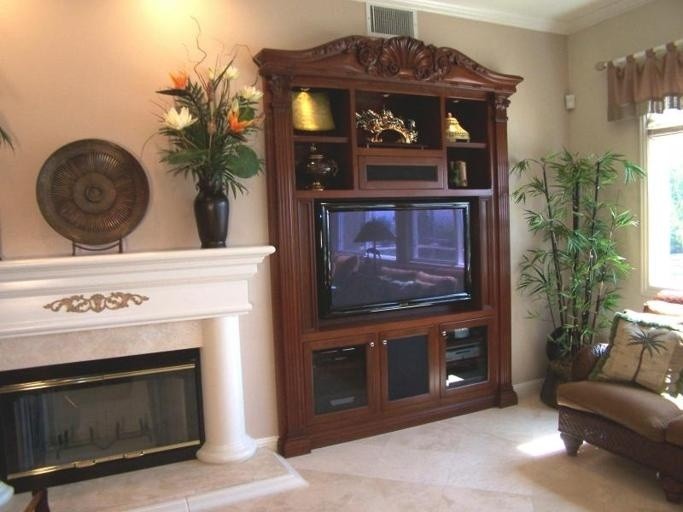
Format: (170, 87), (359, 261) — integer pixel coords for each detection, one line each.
(506, 147), (656, 411)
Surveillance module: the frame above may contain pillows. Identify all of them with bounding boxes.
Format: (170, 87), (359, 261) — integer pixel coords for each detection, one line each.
(586, 309), (683, 399)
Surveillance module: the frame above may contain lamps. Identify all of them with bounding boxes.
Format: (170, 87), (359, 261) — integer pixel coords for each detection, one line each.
(352, 217), (396, 264)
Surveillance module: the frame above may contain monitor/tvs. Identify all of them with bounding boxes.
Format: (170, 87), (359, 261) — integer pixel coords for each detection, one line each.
(317, 202), (472, 319)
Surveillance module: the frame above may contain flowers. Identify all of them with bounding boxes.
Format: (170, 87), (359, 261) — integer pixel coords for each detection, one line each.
(140, 21), (267, 197)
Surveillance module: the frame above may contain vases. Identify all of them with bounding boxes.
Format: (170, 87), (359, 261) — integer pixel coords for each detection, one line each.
(193, 180), (232, 252)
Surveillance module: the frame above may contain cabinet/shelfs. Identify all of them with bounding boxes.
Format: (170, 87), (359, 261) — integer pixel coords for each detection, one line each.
(250, 32), (529, 463)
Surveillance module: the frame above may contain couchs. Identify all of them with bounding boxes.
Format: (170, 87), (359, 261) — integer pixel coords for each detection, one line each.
(332, 251), (466, 310)
(555, 290), (682, 508)
